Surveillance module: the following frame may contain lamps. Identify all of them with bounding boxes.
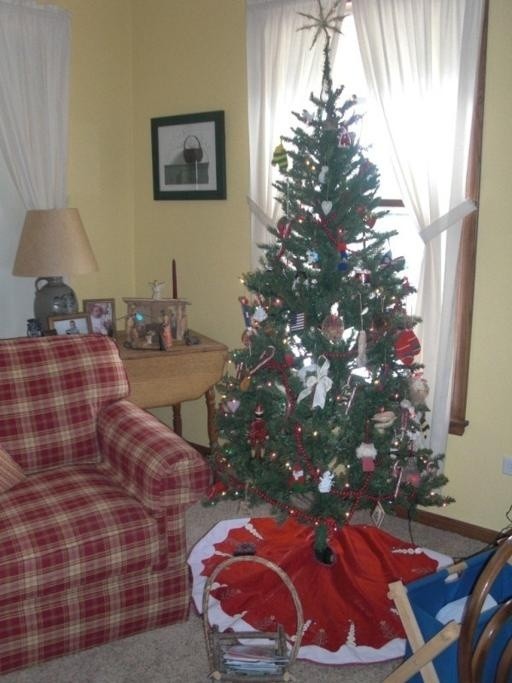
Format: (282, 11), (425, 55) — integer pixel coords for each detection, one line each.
(12, 207), (99, 331)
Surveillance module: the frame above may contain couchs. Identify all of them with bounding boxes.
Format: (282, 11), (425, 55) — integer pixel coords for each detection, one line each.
(0, 333), (212, 675)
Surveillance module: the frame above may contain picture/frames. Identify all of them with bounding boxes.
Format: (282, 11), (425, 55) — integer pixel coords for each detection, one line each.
(48, 313), (93, 335)
(150, 111), (227, 200)
(83, 298), (116, 337)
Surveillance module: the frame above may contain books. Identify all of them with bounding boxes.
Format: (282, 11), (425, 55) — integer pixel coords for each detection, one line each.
(220, 645), (289, 674)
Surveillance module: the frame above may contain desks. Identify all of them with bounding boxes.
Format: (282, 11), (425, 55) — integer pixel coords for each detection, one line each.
(115, 330), (228, 455)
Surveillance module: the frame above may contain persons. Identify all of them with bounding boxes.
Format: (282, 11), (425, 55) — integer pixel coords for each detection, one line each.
(90, 305), (108, 335)
(66, 320), (79, 334)
